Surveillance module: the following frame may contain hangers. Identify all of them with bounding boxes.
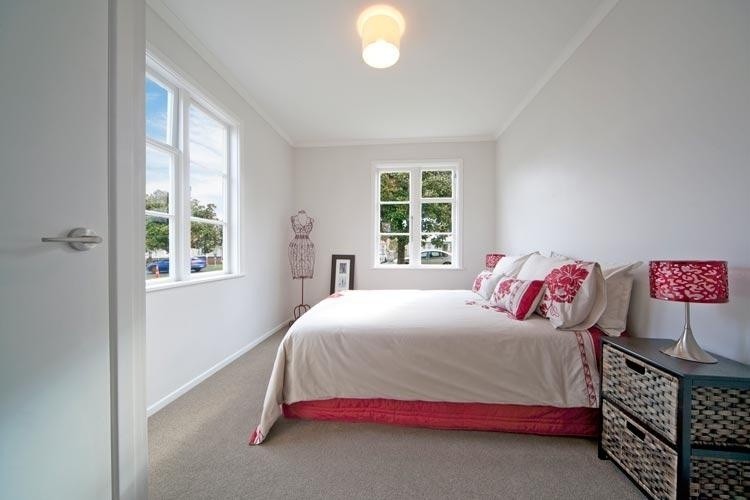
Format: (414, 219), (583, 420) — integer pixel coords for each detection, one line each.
(289, 210), (315, 326)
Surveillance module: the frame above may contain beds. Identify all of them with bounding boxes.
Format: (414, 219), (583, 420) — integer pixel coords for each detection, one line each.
(248, 250), (641, 446)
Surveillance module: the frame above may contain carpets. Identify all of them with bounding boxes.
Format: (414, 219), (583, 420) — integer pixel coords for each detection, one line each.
(149, 320), (650, 499)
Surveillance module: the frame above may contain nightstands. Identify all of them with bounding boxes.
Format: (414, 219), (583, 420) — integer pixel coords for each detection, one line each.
(598, 338), (750, 500)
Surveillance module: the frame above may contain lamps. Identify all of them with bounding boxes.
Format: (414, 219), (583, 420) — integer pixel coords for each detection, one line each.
(355, 4), (405, 71)
(650, 259), (729, 363)
(486, 253), (504, 271)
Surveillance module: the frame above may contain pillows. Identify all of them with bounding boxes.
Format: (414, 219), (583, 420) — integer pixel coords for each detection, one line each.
(490, 276), (546, 319)
(472, 271), (505, 300)
(517, 251), (607, 332)
(594, 260), (644, 336)
(493, 249), (540, 278)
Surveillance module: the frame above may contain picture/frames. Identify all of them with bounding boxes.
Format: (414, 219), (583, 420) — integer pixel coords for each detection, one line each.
(330, 255), (355, 295)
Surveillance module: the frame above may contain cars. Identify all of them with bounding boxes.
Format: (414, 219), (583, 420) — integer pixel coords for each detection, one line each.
(147, 256), (169, 274)
(394, 250), (451, 265)
(192, 256), (205, 272)
